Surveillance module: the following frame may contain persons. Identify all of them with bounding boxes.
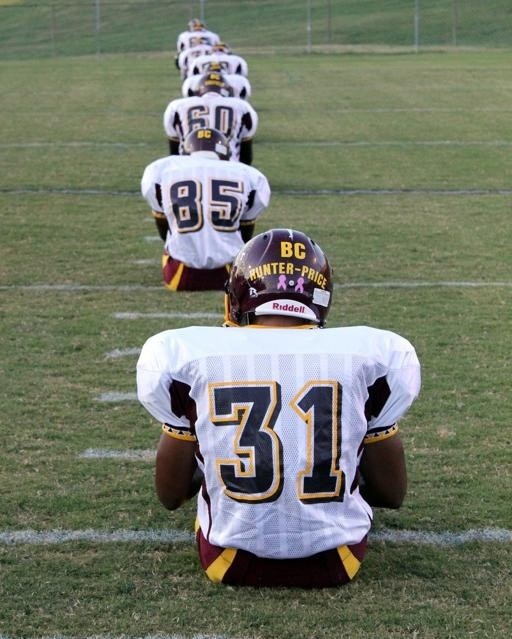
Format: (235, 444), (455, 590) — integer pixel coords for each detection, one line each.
(178, 34), (216, 70)
(162, 72), (258, 165)
(176, 17), (220, 53)
(181, 62), (252, 101)
(137, 127), (272, 292)
(132, 227), (422, 591)
(186, 42), (248, 78)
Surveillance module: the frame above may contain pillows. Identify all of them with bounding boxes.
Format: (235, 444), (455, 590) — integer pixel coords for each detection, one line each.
(198, 72), (232, 99)
(205, 63), (227, 74)
(181, 126), (231, 161)
(212, 42), (232, 55)
(222, 226), (336, 329)
(186, 18), (206, 32)
